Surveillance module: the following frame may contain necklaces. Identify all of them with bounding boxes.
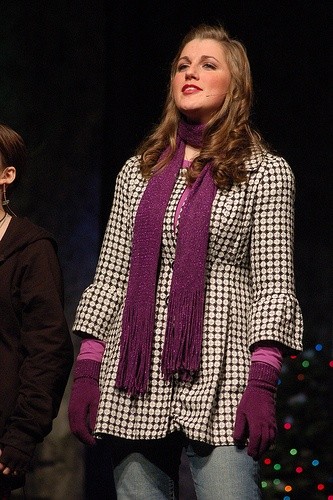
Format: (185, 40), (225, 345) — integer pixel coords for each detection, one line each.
(0, 211), (7, 227)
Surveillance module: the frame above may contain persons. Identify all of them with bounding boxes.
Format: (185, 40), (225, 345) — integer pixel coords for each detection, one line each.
(0, 123), (76, 499)
(67, 27), (304, 500)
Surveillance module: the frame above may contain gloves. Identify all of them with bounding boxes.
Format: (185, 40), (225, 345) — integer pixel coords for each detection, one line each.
(68, 358), (99, 445)
(232, 362), (280, 459)
